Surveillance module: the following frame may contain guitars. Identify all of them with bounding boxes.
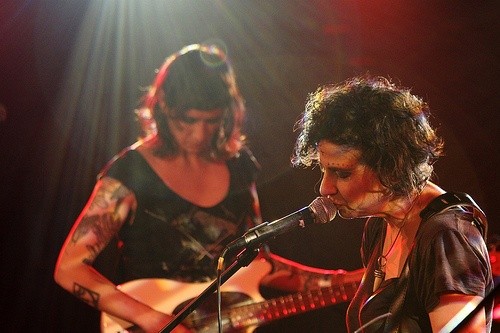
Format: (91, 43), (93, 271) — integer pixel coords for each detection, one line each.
(100, 243), (500, 333)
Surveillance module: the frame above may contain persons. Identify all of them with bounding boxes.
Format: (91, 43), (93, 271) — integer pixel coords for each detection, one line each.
(51, 42), (365, 333)
(286, 74), (496, 333)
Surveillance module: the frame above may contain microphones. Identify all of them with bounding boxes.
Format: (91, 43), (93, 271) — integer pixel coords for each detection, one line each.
(226, 196), (337, 253)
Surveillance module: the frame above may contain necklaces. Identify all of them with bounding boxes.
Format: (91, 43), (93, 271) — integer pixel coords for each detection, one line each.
(369, 204), (414, 293)
